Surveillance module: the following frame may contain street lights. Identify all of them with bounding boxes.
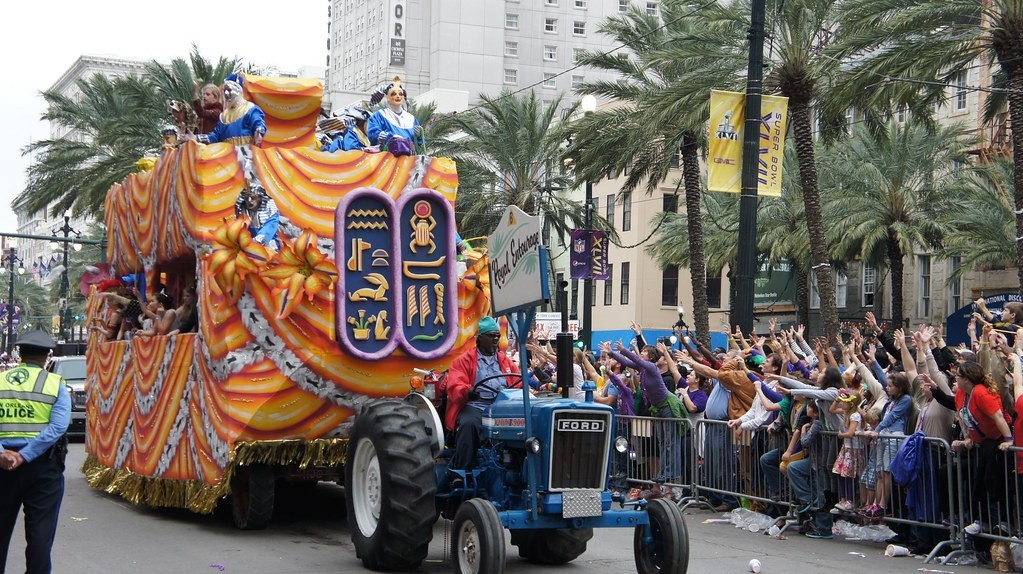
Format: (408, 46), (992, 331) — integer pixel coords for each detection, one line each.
(564, 93), (599, 354)
(0, 247), (25, 357)
(52, 216), (83, 340)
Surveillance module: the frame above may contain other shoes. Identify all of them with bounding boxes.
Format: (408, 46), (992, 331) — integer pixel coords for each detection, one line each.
(452, 474), (471, 488)
(700, 498), (738, 512)
(608, 482), (687, 506)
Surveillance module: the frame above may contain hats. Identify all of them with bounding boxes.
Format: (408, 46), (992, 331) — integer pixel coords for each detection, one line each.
(586, 353), (595, 366)
(954, 347), (977, 362)
(477, 316), (500, 335)
(15, 331), (57, 356)
(225, 74), (244, 87)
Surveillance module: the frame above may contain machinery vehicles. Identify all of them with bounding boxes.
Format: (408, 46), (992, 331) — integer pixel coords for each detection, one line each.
(342, 277), (690, 574)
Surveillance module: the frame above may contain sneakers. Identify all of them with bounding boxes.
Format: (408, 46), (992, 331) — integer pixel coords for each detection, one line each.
(858, 503), (889, 519)
(830, 500), (855, 514)
(964, 520), (990, 535)
(992, 521), (1014, 536)
(796, 493), (819, 513)
(805, 526), (834, 539)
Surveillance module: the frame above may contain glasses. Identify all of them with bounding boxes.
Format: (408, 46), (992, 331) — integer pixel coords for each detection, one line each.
(954, 373), (961, 377)
(486, 333), (501, 338)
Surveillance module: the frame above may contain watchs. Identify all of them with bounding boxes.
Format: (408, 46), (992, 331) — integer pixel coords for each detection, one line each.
(697, 343), (702, 348)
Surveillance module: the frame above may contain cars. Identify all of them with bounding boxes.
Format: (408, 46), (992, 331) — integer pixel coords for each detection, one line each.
(43, 355), (88, 440)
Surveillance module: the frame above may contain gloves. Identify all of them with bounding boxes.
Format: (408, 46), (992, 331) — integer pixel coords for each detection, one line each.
(467, 387), (481, 402)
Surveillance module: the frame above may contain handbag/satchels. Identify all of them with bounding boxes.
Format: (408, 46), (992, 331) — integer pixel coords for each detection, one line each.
(386, 136), (411, 157)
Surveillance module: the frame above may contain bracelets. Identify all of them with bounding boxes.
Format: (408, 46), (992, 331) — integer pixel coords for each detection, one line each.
(544, 352), (548, 357)
(689, 360), (696, 368)
(980, 341), (988, 345)
(916, 347), (923, 350)
(156, 317), (161, 320)
(924, 349), (931, 355)
(1006, 437), (1014, 440)
(835, 399), (839, 403)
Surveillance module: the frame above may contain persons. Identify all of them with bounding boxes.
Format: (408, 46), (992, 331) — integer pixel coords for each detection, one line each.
(180, 73), (267, 146)
(321, 76), (423, 156)
(0, 347), (19, 372)
(193, 77), (223, 135)
(0, 330), (71, 574)
(444, 316), (523, 487)
(91, 280), (199, 343)
(162, 100), (197, 148)
(507, 298), (1023, 564)
(242, 183), (281, 253)
